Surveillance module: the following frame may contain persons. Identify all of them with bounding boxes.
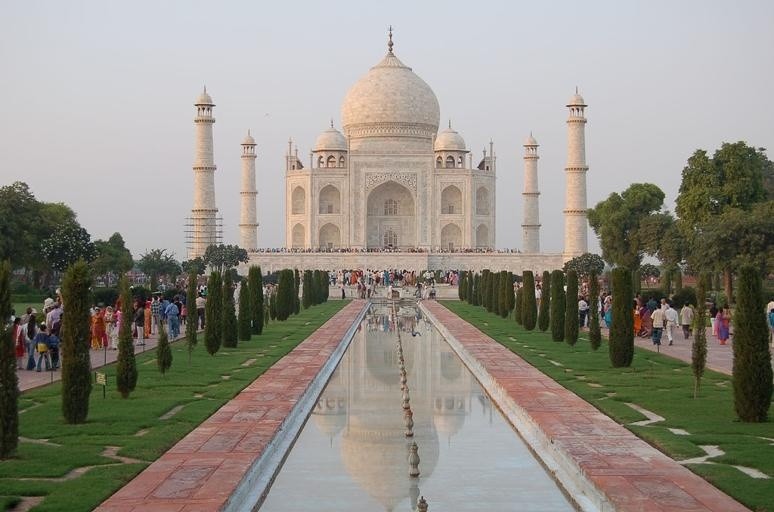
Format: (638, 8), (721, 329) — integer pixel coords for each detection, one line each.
(246, 271), (541, 300)
(578, 290), (774, 345)
(247, 247), (521, 253)
(9, 271), (208, 372)
